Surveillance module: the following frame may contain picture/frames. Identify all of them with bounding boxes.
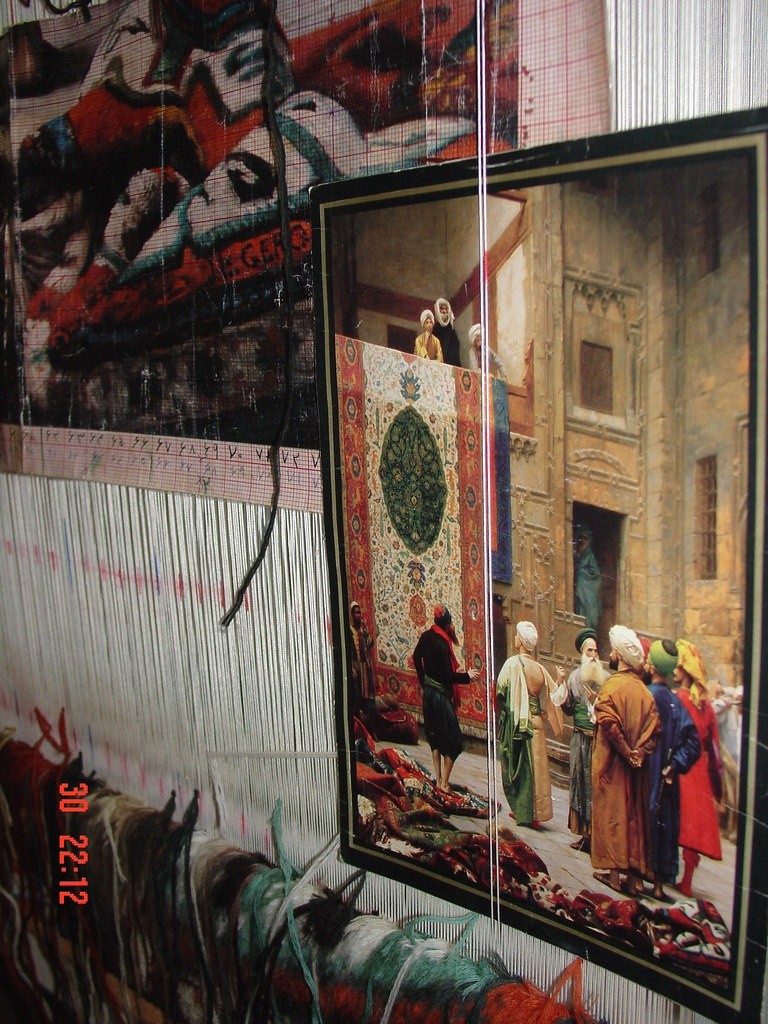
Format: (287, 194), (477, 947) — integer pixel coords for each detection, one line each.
(310, 108), (768, 1023)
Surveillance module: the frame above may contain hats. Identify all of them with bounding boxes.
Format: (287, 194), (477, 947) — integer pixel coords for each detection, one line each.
(609, 625), (651, 671)
(433, 604), (454, 625)
(574, 628), (600, 652)
(647, 639), (681, 676)
(517, 621), (539, 652)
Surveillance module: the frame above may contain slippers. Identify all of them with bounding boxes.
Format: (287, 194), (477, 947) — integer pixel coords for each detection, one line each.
(593, 871), (615, 889)
(569, 840), (592, 851)
(620, 878), (626, 890)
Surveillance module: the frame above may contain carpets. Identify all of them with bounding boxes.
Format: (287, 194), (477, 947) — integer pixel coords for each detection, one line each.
(334, 331), (734, 975)
